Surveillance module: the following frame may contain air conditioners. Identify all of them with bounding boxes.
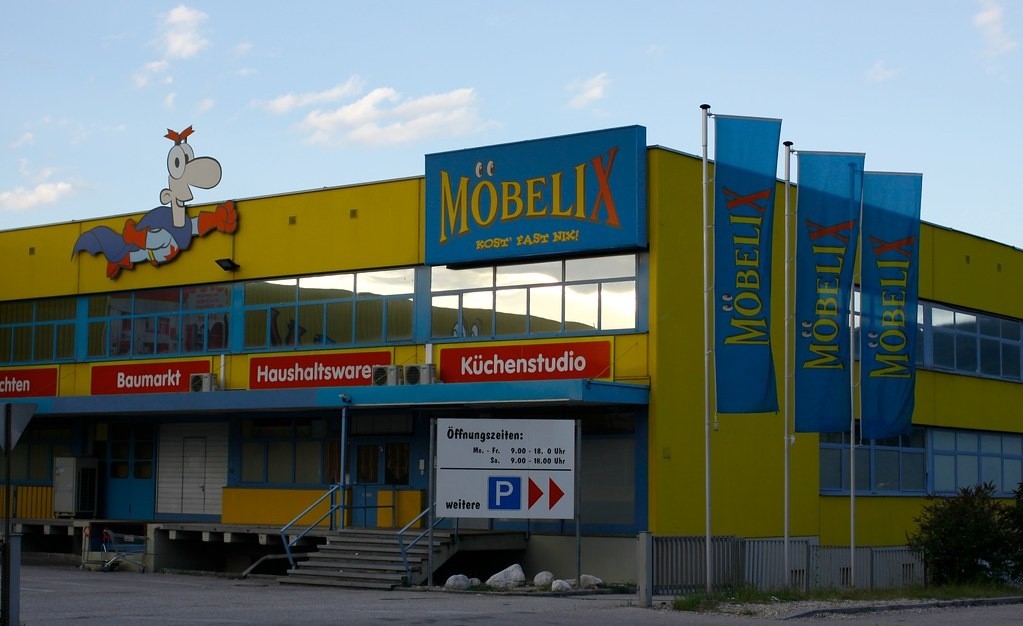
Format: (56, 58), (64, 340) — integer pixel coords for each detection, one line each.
(404, 364), (435, 385)
(189, 373), (218, 392)
(372, 365), (402, 385)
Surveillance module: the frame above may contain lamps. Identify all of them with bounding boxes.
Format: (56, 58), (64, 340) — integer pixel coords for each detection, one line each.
(214, 258), (240, 272)
